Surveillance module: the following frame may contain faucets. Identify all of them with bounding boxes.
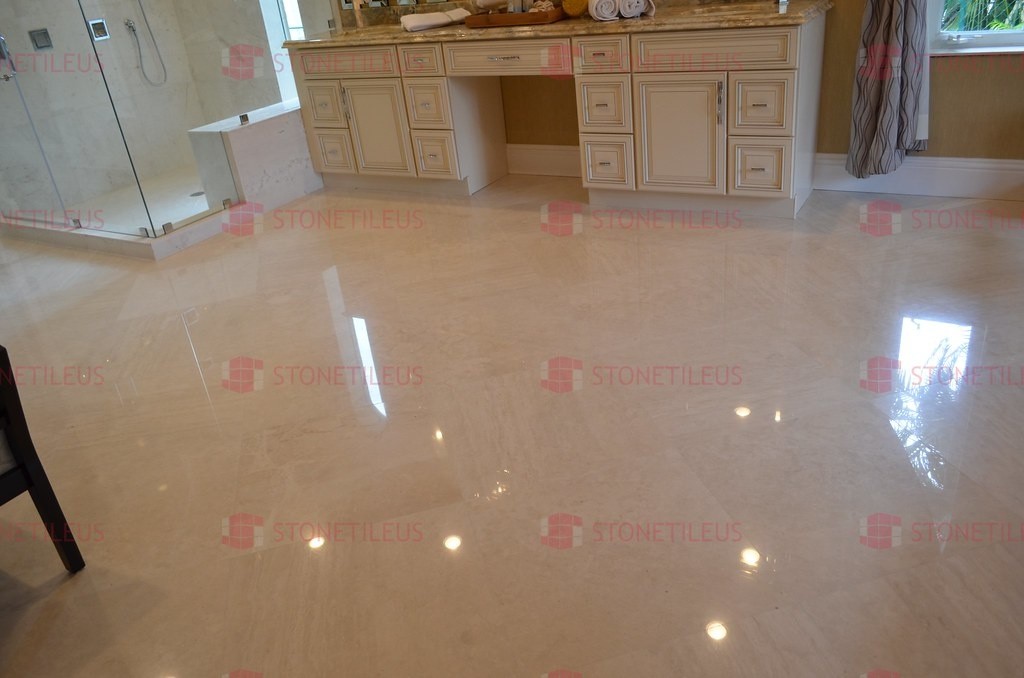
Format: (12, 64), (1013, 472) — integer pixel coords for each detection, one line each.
(407, 1), (416, 13)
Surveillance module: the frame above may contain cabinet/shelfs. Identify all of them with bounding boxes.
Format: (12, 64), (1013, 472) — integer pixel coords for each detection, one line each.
(282, 10), (827, 220)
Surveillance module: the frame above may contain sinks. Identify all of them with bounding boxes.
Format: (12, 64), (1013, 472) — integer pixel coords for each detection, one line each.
(334, 26), (405, 35)
(679, 5), (778, 15)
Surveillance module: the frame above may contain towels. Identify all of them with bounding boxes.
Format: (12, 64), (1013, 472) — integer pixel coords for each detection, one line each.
(399, 7), (471, 31)
(563, 0), (656, 21)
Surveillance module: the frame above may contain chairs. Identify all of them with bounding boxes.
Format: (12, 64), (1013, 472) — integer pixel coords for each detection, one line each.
(0, 342), (86, 574)
